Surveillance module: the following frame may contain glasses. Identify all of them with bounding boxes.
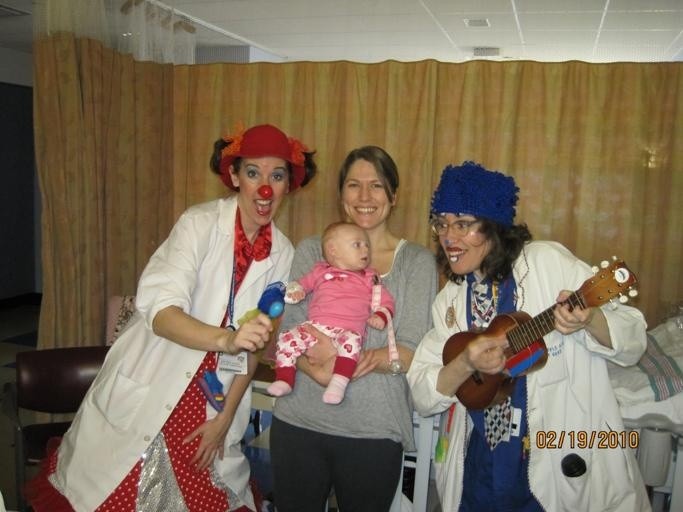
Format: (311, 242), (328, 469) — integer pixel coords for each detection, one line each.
(429, 217), (482, 237)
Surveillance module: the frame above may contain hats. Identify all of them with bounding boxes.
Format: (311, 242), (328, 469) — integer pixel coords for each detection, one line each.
(431, 162), (520, 227)
(219, 125), (307, 194)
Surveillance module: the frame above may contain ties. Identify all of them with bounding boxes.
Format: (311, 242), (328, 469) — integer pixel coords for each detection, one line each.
(470, 277), (512, 454)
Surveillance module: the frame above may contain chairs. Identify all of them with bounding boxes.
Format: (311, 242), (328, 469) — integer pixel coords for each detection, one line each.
(13, 345), (114, 512)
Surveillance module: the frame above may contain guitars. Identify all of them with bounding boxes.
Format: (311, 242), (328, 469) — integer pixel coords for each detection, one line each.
(442, 254), (639, 410)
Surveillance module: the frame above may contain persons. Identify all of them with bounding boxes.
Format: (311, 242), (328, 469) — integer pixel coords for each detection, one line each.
(268, 147), (437, 512)
(20, 124), (318, 512)
(267, 221), (394, 404)
(406, 162), (652, 512)
